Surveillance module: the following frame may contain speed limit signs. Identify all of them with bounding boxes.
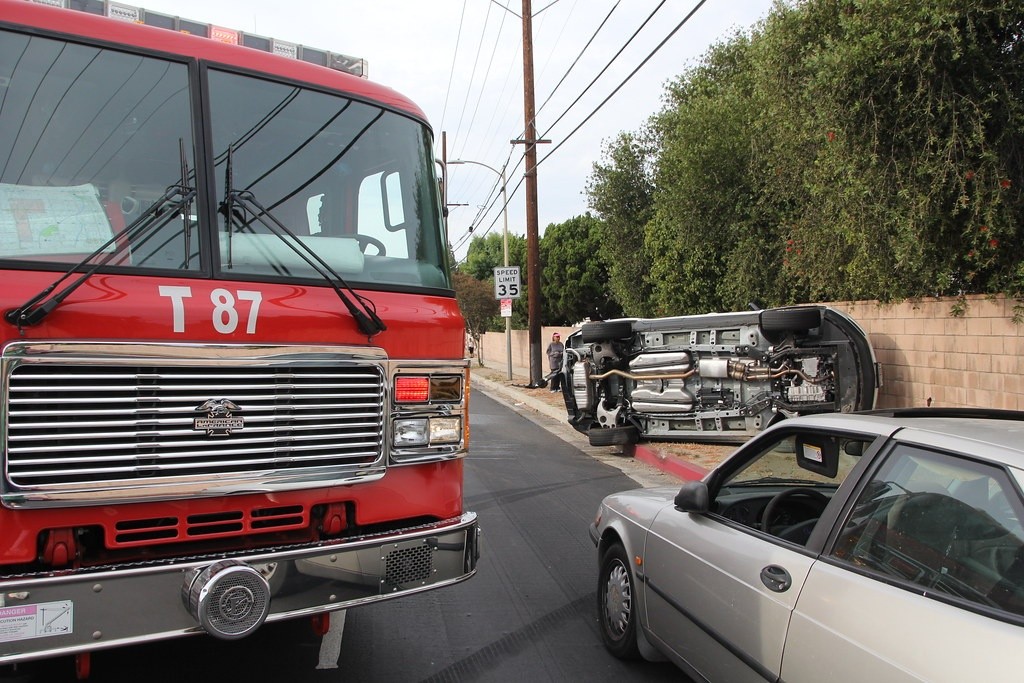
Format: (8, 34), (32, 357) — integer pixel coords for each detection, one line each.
(494, 267), (520, 300)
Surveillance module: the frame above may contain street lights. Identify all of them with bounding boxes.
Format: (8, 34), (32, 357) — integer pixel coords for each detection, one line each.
(446, 160), (513, 382)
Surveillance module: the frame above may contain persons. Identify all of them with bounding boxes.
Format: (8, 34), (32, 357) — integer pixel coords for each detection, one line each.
(468, 333), (474, 358)
(546, 333), (564, 394)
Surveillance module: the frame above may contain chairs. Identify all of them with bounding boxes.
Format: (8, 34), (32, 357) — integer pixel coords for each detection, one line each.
(886, 483), (956, 551)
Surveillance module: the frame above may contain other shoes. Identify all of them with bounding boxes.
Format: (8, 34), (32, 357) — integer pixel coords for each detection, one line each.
(557, 389), (561, 392)
(549, 389), (556, 394)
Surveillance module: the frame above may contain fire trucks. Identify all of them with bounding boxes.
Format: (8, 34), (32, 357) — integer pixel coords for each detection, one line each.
(0, 0), (488, 666)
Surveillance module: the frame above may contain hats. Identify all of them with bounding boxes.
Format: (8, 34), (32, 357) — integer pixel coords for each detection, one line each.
(553, 333), (560, 336)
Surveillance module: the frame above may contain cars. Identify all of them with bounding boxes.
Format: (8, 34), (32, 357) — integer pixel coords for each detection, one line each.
(588, 407), (1023, 683)
(554, 304), (883, 447)
